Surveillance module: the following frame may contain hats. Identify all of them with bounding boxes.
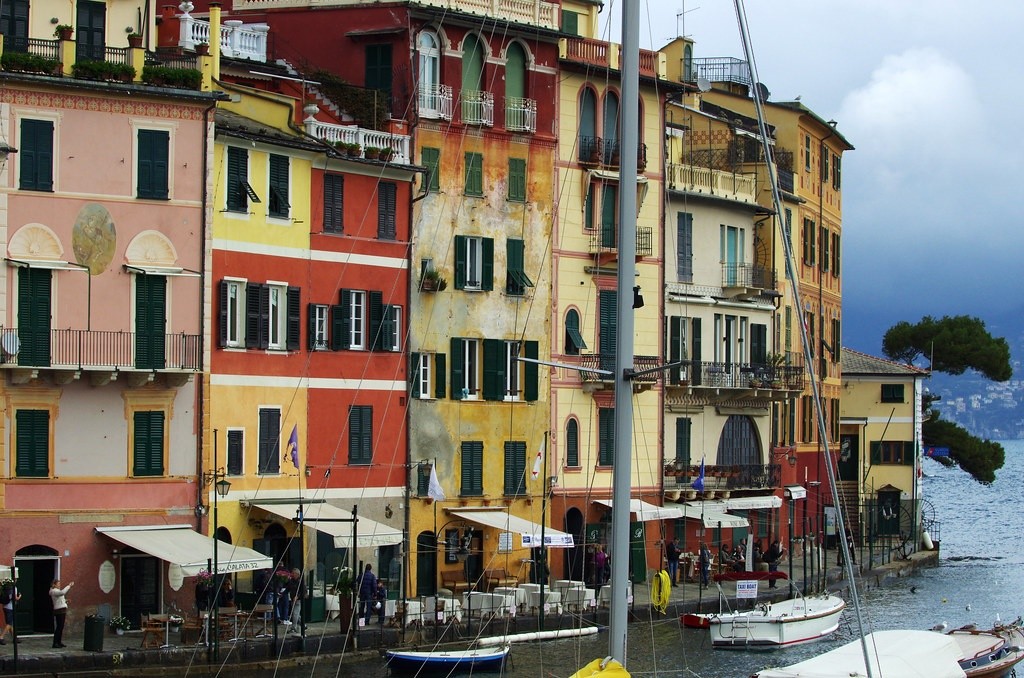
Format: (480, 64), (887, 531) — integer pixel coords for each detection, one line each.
(292, 568), (300, 575)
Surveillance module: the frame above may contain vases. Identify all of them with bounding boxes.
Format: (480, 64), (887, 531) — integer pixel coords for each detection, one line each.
(170, 626), (179, 632)
(117, 628), (124, 635)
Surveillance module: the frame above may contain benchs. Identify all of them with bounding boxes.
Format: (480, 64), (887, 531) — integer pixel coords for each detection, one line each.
(441, 570), (477, 592)
(484, 567), (519, 587)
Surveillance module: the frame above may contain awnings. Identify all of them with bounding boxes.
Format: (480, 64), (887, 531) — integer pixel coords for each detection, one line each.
(93, 523), (274, 577)
(239, 499), (405, 548)
(590, 498), (684, 522)
(668, 495), (783, 528)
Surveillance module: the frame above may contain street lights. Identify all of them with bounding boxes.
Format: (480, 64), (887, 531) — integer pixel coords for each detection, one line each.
(203, 429), (231, 663)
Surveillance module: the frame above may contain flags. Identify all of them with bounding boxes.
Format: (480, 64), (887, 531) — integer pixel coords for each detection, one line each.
(427, 459), (446, 502)
(283, 423), (299, 469)
(690, 458), (704, 494)
(531, 435), (545, 480)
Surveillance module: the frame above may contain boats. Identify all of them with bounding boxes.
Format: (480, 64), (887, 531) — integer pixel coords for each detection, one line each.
(187, 0), (1024, 677)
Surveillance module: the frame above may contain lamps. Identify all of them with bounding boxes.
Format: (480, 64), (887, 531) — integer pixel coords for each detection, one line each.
(770, 442), (797, 468)
(203, 467), (231, 498)
(407, 458), (432, 477)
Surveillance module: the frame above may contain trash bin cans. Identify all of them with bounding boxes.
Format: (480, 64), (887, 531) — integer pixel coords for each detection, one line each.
(84, 614), (107, 651)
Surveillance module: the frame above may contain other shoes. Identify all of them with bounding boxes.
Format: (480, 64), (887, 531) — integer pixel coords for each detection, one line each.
(284, 620), (292, 625)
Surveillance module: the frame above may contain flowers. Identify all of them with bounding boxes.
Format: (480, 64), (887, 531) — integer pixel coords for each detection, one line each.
(192, 567), (211, 588)
(169, 614), (185, 626)
(109, 616), (131, 630)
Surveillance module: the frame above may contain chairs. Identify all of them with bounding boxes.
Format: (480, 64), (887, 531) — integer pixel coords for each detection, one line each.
(140, 604), (273, 650)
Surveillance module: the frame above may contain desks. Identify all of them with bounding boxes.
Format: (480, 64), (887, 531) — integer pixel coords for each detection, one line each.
(152, 613), (251, 644)
(326, 580), (632, 626)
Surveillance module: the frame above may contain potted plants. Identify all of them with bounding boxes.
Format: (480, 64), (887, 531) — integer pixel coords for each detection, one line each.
(53, 24), (209, 91)
(748, 379), (781, 389)
(422, 271), (440, 288)
(665, 465), (740, 478)
(321, 137), (394, 162)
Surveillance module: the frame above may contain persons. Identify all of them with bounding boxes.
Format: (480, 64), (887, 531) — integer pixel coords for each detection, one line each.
(768, 541), (784, 589)
(442, 506), (575, 548)
(358, 564), (377, 625)
(536, 550), (550, 585)
(370, 578), (386, 616)
(262, 561), (293, 626)
(722, 538), (762, 572)
(666, 537), (682, 588)
(49, 578), (75, 648)
(195, 579), (234, 617)
(836, 523), (858, 566)
(701, 542), (710, 590)
(591, 545), (610, 591)
(0, 578), (23, 645)
(279, 568), (308, 634)
